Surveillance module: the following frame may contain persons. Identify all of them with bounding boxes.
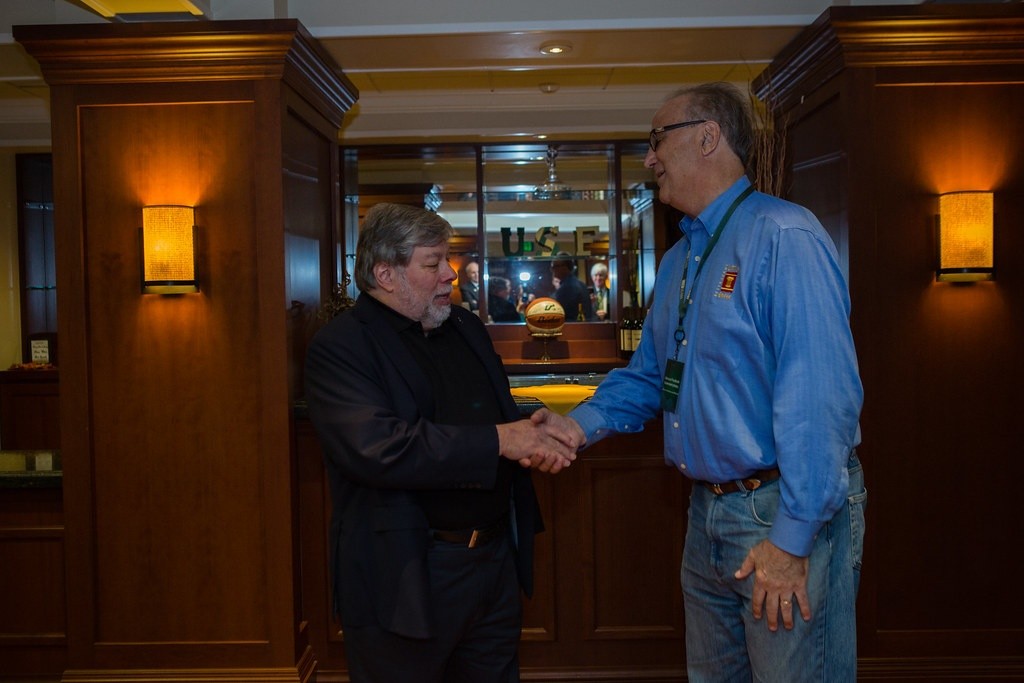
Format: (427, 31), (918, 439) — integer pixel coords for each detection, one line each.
(304, 204), (577, 683)
(526, 84), (869, 683)
(549, 278), (561, 299)
(587, 263), (610, 321)
(459, 262), (481, 310)
(552, 251), (586, 322)
(489, 277), (523, 322)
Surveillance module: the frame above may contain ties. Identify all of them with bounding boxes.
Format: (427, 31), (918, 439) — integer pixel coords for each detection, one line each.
(596, 290), (603, 310)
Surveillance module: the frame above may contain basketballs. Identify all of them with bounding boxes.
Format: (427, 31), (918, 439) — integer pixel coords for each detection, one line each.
(525, 296), (566, 334)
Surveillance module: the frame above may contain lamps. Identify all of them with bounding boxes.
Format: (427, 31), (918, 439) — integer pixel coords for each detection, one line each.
(137, 205), (200, 298)
(932, 189), (998, 287)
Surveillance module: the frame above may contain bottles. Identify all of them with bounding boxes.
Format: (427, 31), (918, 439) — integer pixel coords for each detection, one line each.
(577, 304), (584, 321)
(617, 308), (632, 357)
(632, 309), (644, 359)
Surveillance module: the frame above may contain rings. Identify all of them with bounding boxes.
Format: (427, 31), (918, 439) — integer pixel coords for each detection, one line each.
(781, 600), (790, 605)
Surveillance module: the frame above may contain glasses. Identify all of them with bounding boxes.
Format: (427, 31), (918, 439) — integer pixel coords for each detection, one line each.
(648, 120), (707, 152)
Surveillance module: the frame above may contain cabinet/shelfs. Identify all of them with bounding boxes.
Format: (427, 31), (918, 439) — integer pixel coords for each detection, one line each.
(293, 396), (690, 683)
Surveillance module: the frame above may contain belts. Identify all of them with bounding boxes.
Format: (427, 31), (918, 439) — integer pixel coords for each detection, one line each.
(696, 466), (781, 496)
(429, 527), (502, 551)
